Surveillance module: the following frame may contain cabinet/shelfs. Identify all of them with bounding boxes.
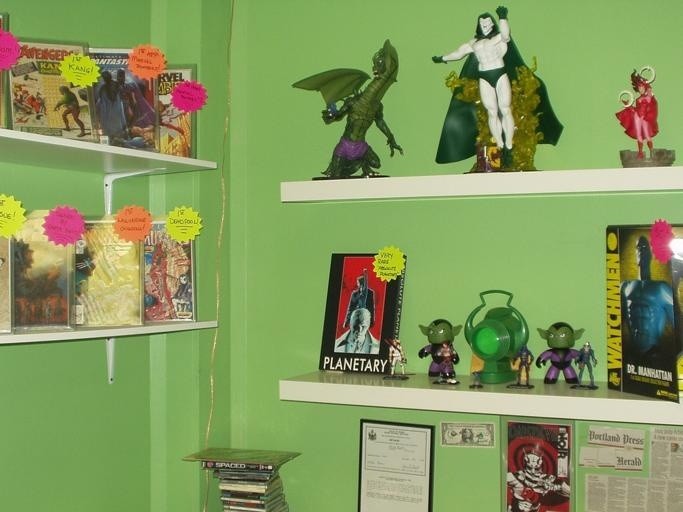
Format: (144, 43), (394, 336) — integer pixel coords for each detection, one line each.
(1, 127), (218, 346)
(275, 162), (683, 426)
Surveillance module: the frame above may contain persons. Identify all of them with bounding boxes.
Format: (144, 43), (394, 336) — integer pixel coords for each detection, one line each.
(436, 343), (456, 385)
(432, 6), (514, 166)
(388, 339), (405, 376)
(334, 308), (380, 354)
(616, 69), (659, 160)
(512, 346), (534, 386)
(576, 342), (598, 387)
(343, 269), (375, 329)
(620, 235), (674, 361)
